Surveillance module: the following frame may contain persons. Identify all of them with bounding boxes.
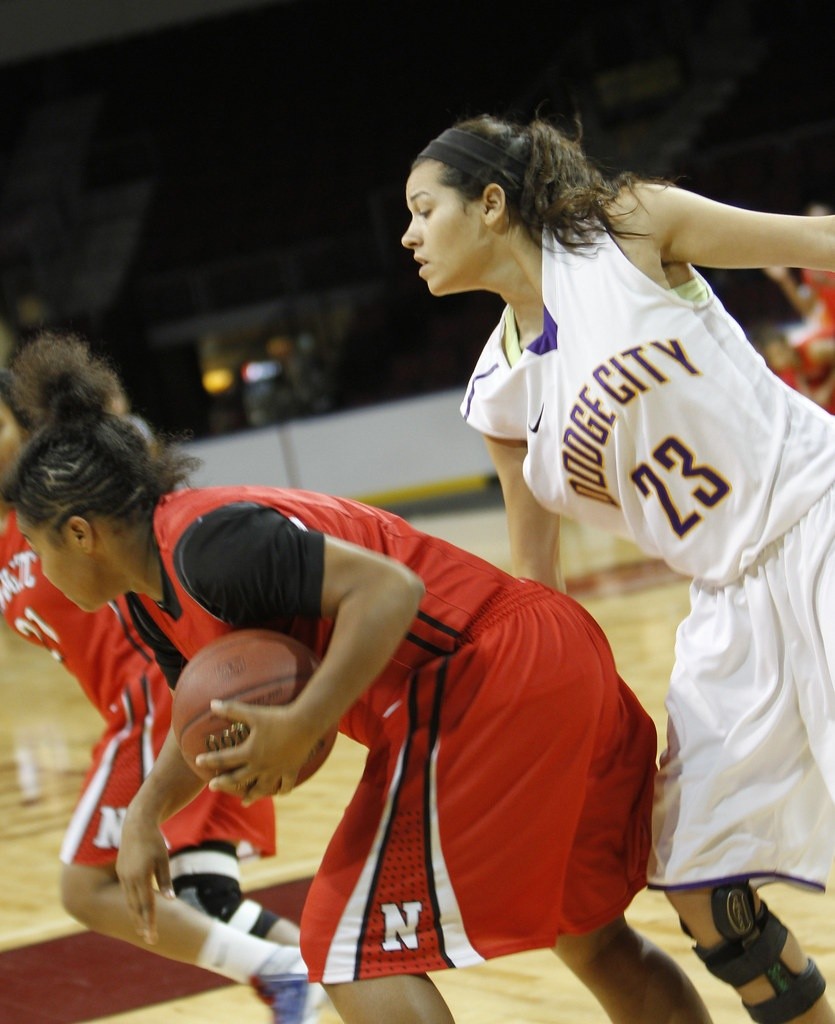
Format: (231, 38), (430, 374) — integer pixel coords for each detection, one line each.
(0, 334), (329, 1024)
(400, 117), (835, 1024)
(5, 409), (715, 1024)
(751, 202), (835, 420)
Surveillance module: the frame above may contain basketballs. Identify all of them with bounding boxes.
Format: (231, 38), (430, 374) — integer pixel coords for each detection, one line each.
(171, 628), (340, 798)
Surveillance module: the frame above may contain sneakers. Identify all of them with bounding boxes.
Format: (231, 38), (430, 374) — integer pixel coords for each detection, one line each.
(248, 942), (328, 1024)
